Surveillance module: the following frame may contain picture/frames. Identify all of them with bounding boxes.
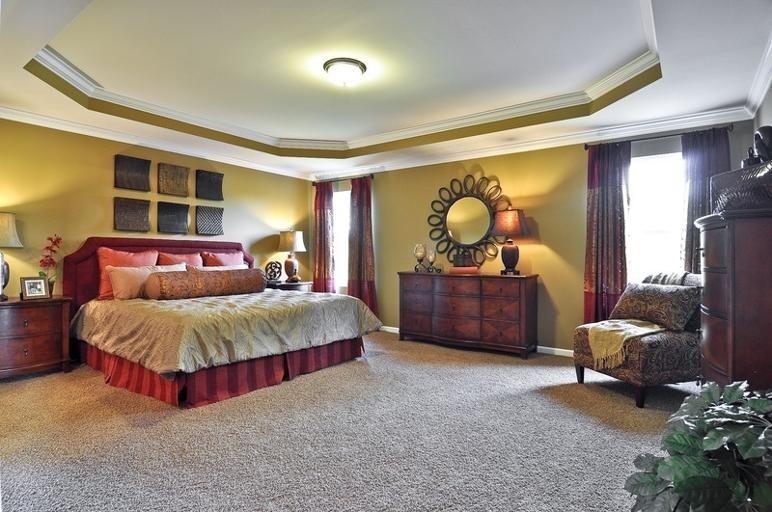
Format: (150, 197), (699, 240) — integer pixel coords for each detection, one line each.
(19, 277), (51, 301)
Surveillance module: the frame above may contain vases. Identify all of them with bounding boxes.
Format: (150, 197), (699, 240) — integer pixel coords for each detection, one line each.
(47, 280), (55, 295)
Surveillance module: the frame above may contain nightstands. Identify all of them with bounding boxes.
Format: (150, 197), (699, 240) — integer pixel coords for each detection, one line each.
(265, 280), (314, 293)
(0, 296), (74, 381)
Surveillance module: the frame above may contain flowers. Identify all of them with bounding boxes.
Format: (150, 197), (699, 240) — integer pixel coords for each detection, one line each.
(37, 233), (65, 280)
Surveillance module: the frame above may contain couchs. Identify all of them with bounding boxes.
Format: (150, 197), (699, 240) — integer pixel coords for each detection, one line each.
(573, 272), (703, 408)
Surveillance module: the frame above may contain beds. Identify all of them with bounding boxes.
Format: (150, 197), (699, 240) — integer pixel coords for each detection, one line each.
(61, 237), (384, 412)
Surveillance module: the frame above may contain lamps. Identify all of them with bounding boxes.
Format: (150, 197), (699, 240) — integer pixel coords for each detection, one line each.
(493, 205), (530, 275)
(322, 57), (367, 84)
(0, 211), (24, 302)
(276, 231), (308, 283)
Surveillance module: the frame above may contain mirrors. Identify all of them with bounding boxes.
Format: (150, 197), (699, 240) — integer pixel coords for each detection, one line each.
(427, 174), (513, 267)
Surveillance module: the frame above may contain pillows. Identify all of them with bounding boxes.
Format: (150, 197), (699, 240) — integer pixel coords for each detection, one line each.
(201, 250), (245, 268)
(104, 262), (187, 301)
(157, 251), (204, 269)
(146, 268), (268, 300)
(608, 281), (701, 332)
(185, 264), (250, 272)
(95, 246), (160, 300)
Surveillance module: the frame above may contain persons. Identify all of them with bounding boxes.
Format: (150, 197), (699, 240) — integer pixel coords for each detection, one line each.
(27, 281), (42, 294)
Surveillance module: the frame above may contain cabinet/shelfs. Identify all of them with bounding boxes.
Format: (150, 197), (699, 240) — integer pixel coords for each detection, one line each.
(693, 209), (772, 393)
(397, 270), (540, 360)
